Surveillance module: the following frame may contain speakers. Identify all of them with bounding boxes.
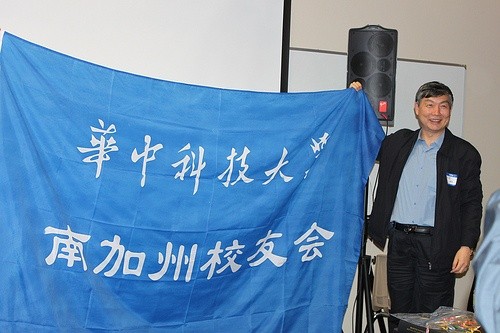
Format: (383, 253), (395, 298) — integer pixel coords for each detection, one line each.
(347, 25), (398, 121)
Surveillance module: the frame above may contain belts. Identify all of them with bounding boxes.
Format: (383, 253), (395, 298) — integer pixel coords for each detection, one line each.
(393, 221), (434, 235)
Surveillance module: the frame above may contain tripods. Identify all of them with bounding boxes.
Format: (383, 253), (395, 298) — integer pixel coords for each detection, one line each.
(355, 178), (386, 333)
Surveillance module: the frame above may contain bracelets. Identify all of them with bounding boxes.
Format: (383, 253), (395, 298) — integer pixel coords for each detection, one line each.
(470, 251), (475, 260)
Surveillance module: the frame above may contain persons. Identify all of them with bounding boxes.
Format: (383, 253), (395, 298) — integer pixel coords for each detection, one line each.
(349, 81), (483, 333)
(471, 188), (500, 333)
